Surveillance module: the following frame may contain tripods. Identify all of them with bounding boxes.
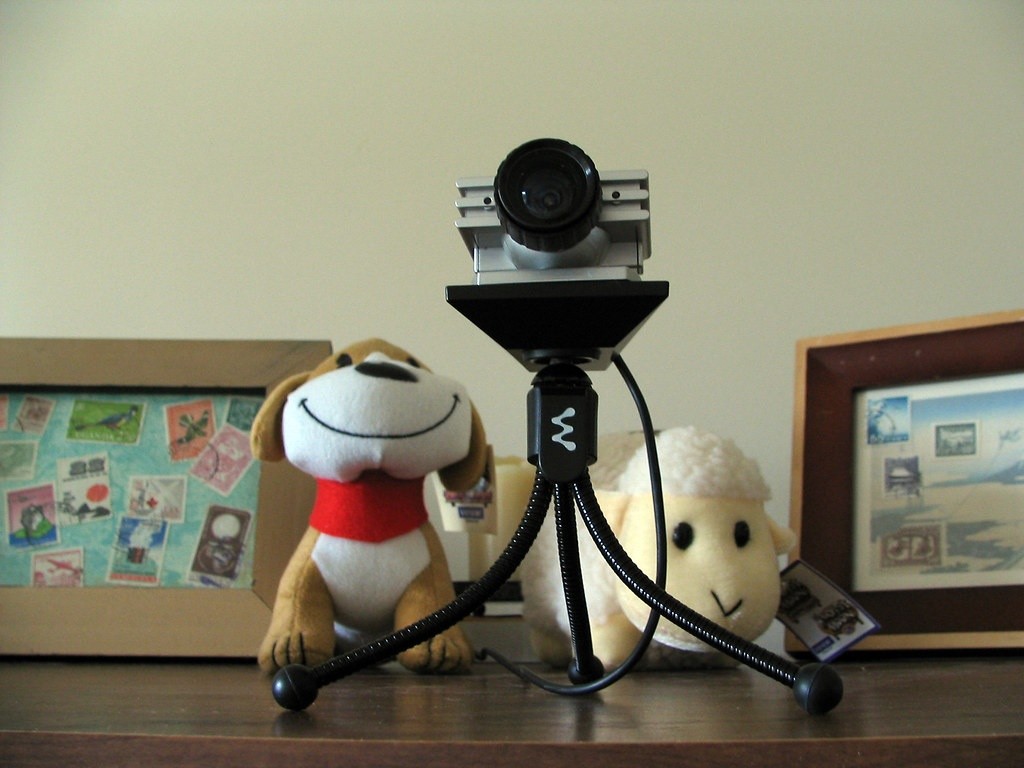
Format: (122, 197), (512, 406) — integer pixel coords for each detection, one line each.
(272, 280), (844, 719)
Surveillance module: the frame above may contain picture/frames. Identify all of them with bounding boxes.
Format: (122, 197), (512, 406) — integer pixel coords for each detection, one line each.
(781, 311), (1023, 655)
(1, 336), (343, 659)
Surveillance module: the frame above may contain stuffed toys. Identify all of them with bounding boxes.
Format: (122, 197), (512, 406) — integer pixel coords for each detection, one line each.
(248, 337), (489, 675)
(519, 425), (797, 673)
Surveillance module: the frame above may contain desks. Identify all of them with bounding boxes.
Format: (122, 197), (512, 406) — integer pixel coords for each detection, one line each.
(0, 642), (1023, 767)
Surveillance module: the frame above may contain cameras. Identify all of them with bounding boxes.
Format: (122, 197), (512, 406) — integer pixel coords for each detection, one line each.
(456, 138), (652, 280)
